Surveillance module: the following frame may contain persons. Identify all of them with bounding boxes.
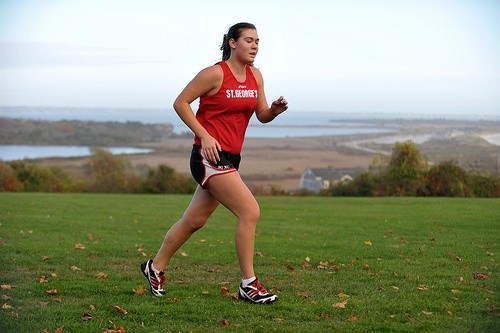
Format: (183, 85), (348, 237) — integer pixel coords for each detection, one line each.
(140, 20), (288, 305)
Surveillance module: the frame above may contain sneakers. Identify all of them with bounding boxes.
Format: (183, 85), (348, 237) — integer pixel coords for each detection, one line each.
(140, 259), (166, 297)
(239, 278), (278, 305)
